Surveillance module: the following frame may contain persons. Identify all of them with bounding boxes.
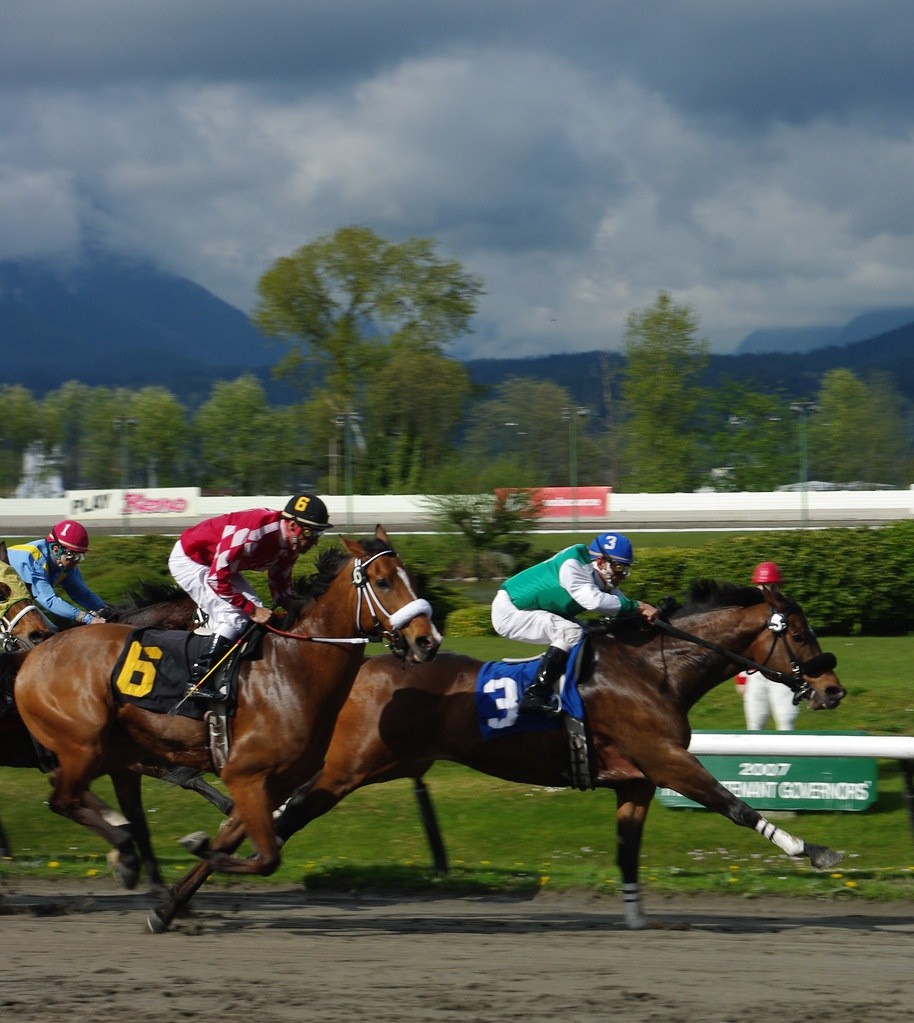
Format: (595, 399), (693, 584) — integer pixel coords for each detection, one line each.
(8, 523), (113, 633)
(490, 528), (664, 721)
(735, 559), (800, 732)
(169, 492), (333, 699)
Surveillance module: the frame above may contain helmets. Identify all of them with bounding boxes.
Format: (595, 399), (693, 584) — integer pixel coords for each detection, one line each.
(280, 494), (333, 530)
(752, 562), (782, 582)
(47, 520), (88, 552)
(587, 533), (633, 566)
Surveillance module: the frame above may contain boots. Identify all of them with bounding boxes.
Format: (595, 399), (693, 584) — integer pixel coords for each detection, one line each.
(181, 633), (227, 698)
(517, 645), (569, 712)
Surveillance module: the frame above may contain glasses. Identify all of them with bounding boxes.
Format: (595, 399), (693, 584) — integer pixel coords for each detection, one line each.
(297, 523), (325, 539)
(610, 562), (631, 577)
(63, 551), (85, 563)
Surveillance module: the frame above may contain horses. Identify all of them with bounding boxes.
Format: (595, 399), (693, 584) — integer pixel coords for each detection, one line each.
(0, 569), (237, 885)
(1, 542), (58, 714)
(145, 576), (848, 933)
(12, 519), (442, 894)
